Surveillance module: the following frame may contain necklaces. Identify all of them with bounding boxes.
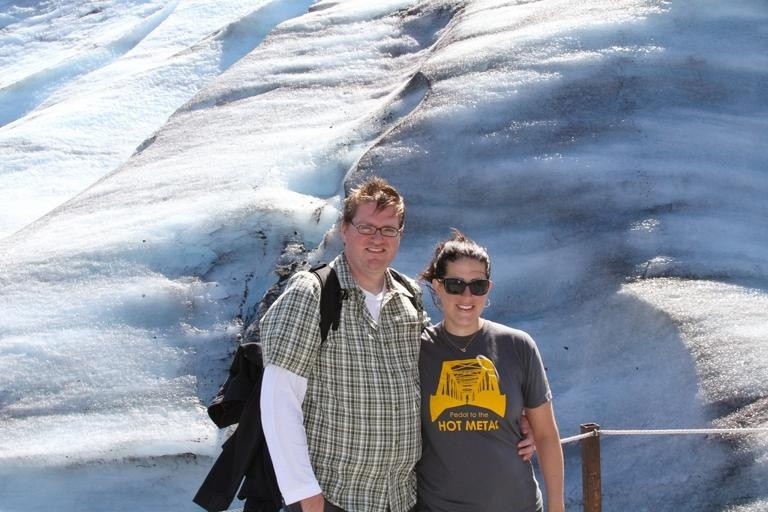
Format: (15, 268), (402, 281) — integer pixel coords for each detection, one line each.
(440, 318), (487, 353)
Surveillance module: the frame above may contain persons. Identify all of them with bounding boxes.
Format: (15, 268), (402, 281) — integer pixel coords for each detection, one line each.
(260, 176), (538, 511)
(413, 225), (567, 511)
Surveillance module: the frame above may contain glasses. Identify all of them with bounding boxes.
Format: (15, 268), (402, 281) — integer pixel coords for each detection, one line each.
(351, 219), (400, 239)
(441, 276), (491, 296)
